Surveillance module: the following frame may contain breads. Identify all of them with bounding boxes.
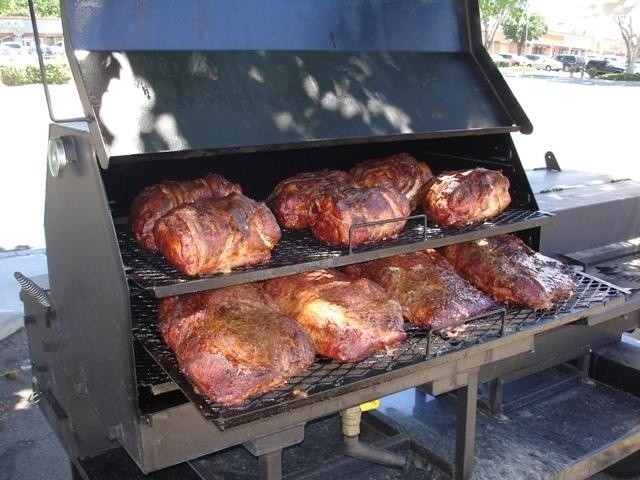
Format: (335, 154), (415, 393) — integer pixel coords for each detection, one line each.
(156, 233), (576, 406)
(129, 152), (511, 278)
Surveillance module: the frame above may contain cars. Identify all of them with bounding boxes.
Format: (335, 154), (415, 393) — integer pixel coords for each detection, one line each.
(491, 54), (625, 75)
(2, 39), (66, 62)
